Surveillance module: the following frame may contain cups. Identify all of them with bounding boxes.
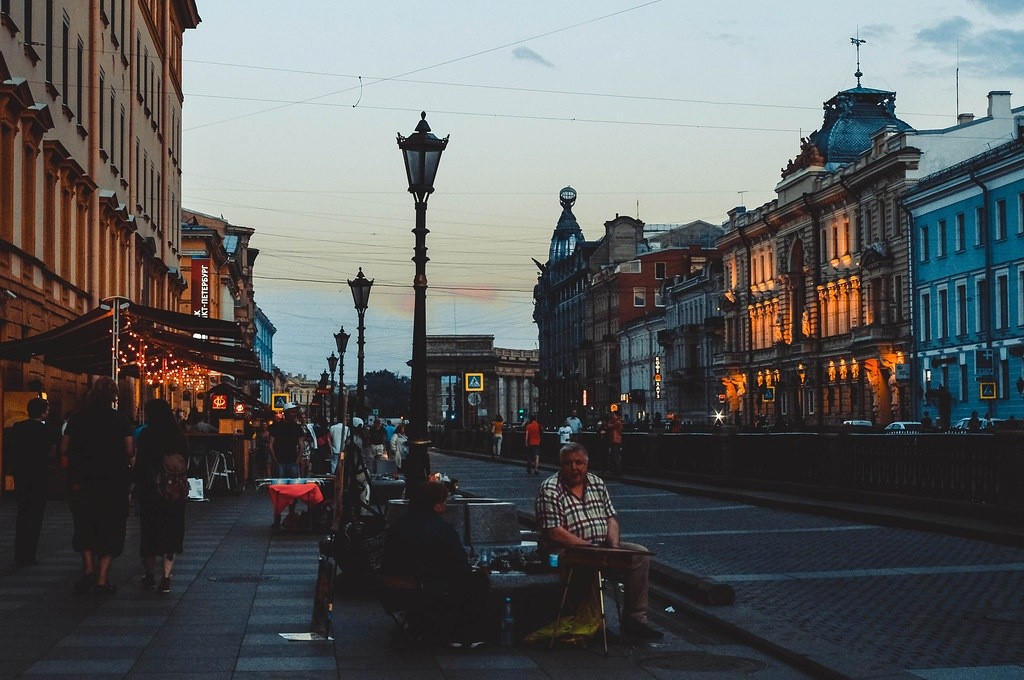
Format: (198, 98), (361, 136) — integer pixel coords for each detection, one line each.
(548, 554), (560, 567)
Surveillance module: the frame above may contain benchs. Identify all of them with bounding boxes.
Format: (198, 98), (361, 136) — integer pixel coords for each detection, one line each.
(383, 498), (559, 590)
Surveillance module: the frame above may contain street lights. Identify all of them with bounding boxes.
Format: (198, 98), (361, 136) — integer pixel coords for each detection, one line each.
(396, 110), (451, 495)
(315, 265), (375, 421)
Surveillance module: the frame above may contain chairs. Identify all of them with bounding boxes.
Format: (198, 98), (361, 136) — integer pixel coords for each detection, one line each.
(374, 575), (447, 653)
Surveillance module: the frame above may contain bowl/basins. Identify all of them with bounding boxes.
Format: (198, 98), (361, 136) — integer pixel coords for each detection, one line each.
(521, 560), (541, 575)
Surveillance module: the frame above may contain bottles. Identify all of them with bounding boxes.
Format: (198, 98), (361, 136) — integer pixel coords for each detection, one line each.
(496, 597), (518, 647)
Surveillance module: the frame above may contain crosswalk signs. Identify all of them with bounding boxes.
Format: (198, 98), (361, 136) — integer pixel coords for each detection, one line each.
(465, 373), (484, 391)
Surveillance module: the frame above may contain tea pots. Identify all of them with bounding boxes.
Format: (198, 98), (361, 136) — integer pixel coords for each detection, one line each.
(499, 559), (510, 573)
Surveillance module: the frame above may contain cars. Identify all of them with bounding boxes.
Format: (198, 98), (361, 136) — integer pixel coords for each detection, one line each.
(953, 417), (1009, 433)
(882, 422), (923, 444)
(843, 419), (873, 435)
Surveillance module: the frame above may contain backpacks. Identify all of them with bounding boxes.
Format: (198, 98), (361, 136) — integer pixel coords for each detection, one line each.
(155, 451), (190, 498)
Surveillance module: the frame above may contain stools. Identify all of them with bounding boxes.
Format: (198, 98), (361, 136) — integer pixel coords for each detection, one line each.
(546, 550), (629, 658)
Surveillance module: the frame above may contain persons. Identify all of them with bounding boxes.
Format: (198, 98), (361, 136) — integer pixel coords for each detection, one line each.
(566, 409), (583, 443)
(921, 410), (932, 426)
(607, 411), (624, 463)
(624, 414), (634, 425)
(60, 374), (136, 598)
(535, 442), (666, 646)
(9, 397), (59, 564)
(346, 416), (409, 481)
(382, 481), (493, 647)
(491, 415), (503, 458)
(967, 411), (994, 432)
(557, 419), (574, 446)
(131, 398), (191, 594)
(174, 405), (219, 433)
(253, 401), (351, 479)
(526, 416), (543, 474)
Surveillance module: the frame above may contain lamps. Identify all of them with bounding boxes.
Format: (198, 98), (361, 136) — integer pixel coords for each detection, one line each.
(197, 392), (204, 399)
(183, 391), (193, 402)
(168, 382), (178, 392)
(153, 381), (159, 388)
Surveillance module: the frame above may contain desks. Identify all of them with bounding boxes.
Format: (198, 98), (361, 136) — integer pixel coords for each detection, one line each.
(184, 433), (243, 491)
(254, 477), (334, 536)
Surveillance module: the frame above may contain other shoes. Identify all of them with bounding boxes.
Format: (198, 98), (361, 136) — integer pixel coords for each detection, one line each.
(75, 580), (97, 592)
(619, 612), (664, 639)
(140, 574), (158, 590)
(15, 556), (39, 566)
(158, 576), (171, 593)
(94, 581), (116, 595)
(534, 471), (539, 475)
(449, 633), (490, 648)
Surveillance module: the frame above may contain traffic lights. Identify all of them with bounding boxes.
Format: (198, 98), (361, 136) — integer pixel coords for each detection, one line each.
(519, 409), (525, 418)
